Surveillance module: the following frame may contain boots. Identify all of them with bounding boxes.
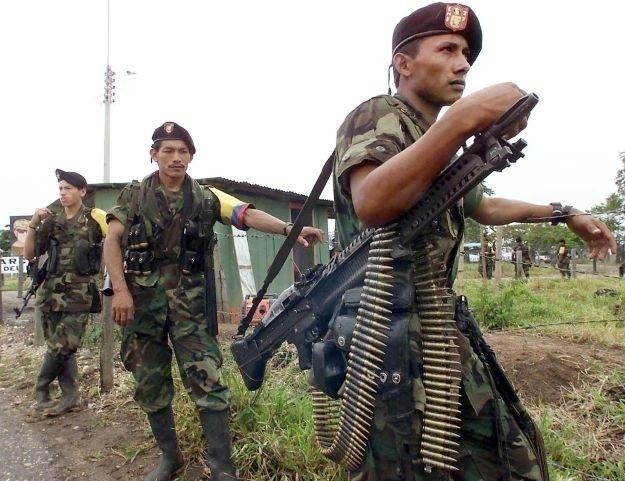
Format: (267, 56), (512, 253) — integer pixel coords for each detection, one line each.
(45, 354), (82, 416)
(198, 408), (238, 481)
(144, 402), (186, 481)
(32, 356), (67, 407)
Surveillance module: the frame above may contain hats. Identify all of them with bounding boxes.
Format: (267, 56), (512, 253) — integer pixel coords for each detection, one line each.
(392, 1), (482, 67)
(56, 168), (88, 188)
(151, 122), (196, 153)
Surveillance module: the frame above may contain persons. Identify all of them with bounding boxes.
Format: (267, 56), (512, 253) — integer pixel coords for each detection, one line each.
(554, 237), (571, 280)
(511, 236), (533, 279)
(331, 1), (620, 479)
(23, 168), (109, 416)
(101, 120), (326, 481)
(477, 237), (493, 279)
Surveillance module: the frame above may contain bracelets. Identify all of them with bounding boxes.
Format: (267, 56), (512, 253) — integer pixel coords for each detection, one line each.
(561, 204), (572, 224)
(283, 221), (294, 236)
(550, 201), (561, 227)
(28, 225), (36, 230)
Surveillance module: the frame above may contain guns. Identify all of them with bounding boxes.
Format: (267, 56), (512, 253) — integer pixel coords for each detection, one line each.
(13, 258), (49, 319)
(230, 93), (539, 399)
